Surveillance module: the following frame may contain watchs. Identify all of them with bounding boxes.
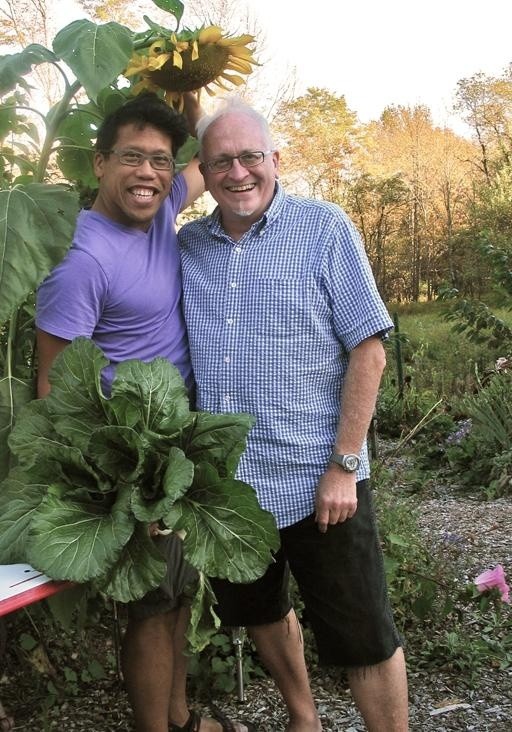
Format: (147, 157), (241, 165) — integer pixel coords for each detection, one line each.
(329, 452), (362, 472)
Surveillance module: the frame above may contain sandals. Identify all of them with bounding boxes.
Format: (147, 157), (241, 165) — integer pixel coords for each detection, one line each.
(163, 704), (256, 731)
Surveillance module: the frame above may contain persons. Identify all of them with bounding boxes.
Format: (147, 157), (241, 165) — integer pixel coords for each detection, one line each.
(32, 89), (250, 732)
(176, 97), (408, 732)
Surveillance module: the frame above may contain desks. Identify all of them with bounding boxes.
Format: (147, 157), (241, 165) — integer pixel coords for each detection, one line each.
(0, 563), (123, 685)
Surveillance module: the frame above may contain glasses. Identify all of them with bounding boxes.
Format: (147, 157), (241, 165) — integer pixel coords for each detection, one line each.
(99, 149), (174, 171)
(199, 150), (272, 172)
